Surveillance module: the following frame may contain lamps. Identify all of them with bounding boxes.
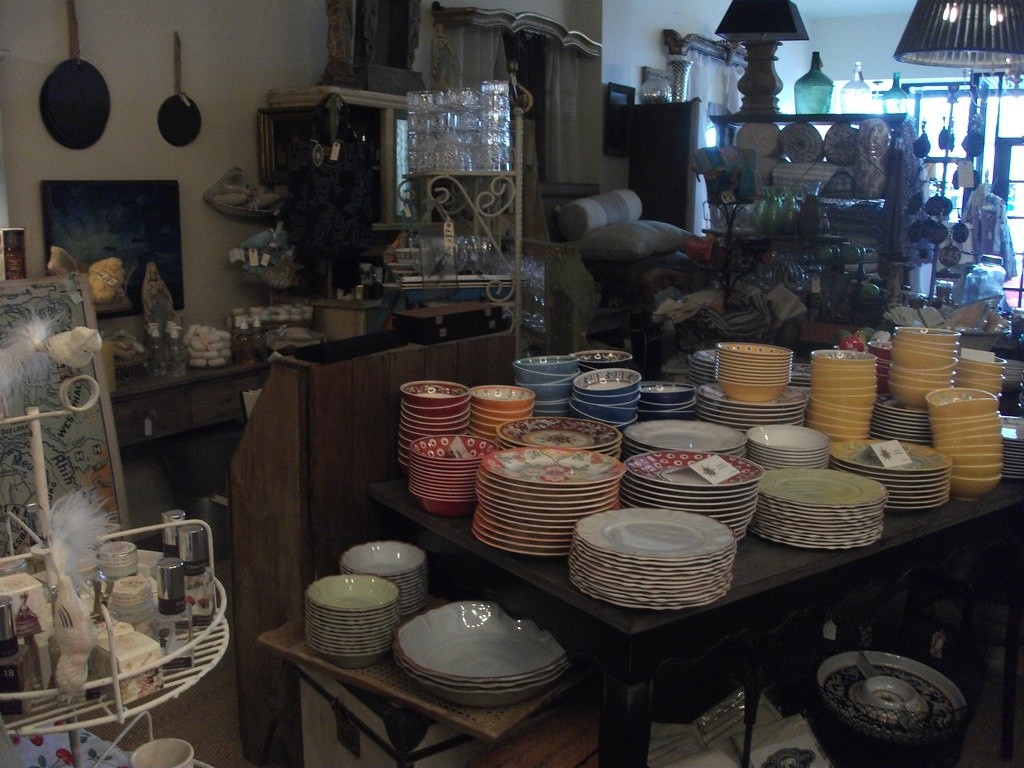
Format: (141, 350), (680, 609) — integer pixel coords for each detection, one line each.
(893, 0), (1024, 72)
(716, 0), (808, 112)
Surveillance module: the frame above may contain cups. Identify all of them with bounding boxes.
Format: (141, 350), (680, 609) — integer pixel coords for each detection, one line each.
(417, 234), (545, 331)
(974, 255), (1007, 303)
(407, 79), (511, 171)
(129, 738), (195, 768)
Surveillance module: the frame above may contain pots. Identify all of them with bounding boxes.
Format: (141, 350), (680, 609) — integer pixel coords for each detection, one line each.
(816, 650), (967, 745)
(156, 31), (203, 148)
(39, 0), (110, 150)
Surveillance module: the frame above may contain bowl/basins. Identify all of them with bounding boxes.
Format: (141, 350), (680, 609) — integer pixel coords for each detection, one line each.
(399, 348), (698, 517)
(394, 248), (420, 264)
(805, 327), (1024, 505)
(713, 343), (794, 403)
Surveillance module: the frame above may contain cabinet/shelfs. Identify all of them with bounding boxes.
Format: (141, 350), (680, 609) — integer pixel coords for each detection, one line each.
(399, 107), (525, 334)
(710, 111), (915, 276)
(107, 359), (270, 448)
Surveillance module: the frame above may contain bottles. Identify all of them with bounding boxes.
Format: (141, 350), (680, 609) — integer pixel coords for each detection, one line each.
(839, 60), (871, 114)
(793, 50), (835, 114)
(881, 71), (909, 114)
(752, 186), (801, 236)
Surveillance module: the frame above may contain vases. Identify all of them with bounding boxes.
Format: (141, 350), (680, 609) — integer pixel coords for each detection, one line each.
(667, 58), (694, 103)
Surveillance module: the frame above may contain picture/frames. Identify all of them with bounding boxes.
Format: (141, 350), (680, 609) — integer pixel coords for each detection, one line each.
(603, 82), (637, 159)
(41, 179), (184, 321)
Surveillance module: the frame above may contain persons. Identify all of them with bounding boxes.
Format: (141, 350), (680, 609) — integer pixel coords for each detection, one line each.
(142, 261), (175, 321)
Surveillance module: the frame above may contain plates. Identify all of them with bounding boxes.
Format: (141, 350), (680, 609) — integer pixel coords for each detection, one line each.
(386, 263), (419, 270)
(397, 324), (1024, 610)
(303, 540), (572, 706)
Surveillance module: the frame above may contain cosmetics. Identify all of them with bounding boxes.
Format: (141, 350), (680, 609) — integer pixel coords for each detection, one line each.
(0, 506), (218, 716)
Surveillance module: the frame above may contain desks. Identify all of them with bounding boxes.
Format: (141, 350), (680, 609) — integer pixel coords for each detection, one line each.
(376, 472), (1024, 767)
(312, 299), (389, 342)
